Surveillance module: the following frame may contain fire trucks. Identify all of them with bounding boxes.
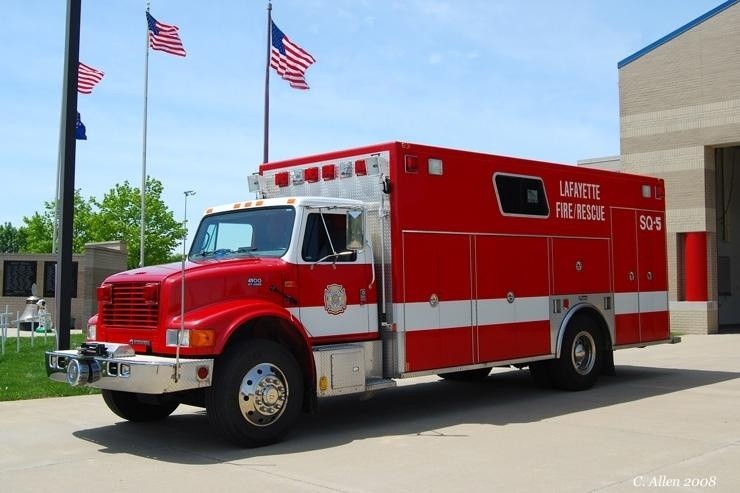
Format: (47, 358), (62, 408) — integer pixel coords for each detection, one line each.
(43, 141), (671, 446)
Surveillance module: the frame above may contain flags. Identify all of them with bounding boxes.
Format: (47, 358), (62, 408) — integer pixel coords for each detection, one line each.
(145, 11), (187, 57)
(77, 61), (106, 95)
(75, 111), (87, 140)
(270, 19), (317, 90)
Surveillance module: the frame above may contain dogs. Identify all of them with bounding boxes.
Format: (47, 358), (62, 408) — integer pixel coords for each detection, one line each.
(36, 299), (52, 331)
